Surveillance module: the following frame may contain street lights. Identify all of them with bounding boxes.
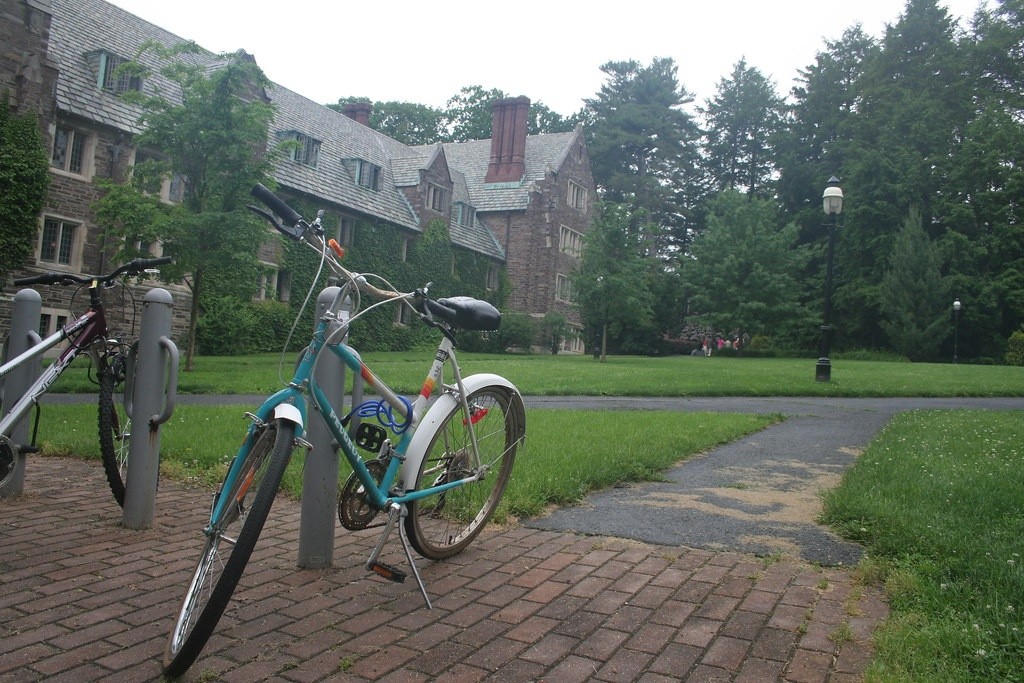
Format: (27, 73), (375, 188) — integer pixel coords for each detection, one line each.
(954, 297), (961, 362)
(816, 174), (843, 380)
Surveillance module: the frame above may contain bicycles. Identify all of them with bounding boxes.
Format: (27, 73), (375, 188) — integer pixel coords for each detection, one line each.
(0, 256), (175, 504)
(162, 183), (527, 677)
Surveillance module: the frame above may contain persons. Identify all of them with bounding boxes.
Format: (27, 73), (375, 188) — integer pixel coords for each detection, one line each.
(718, 337), (739, 350)
(704, 337), (712, 356)
